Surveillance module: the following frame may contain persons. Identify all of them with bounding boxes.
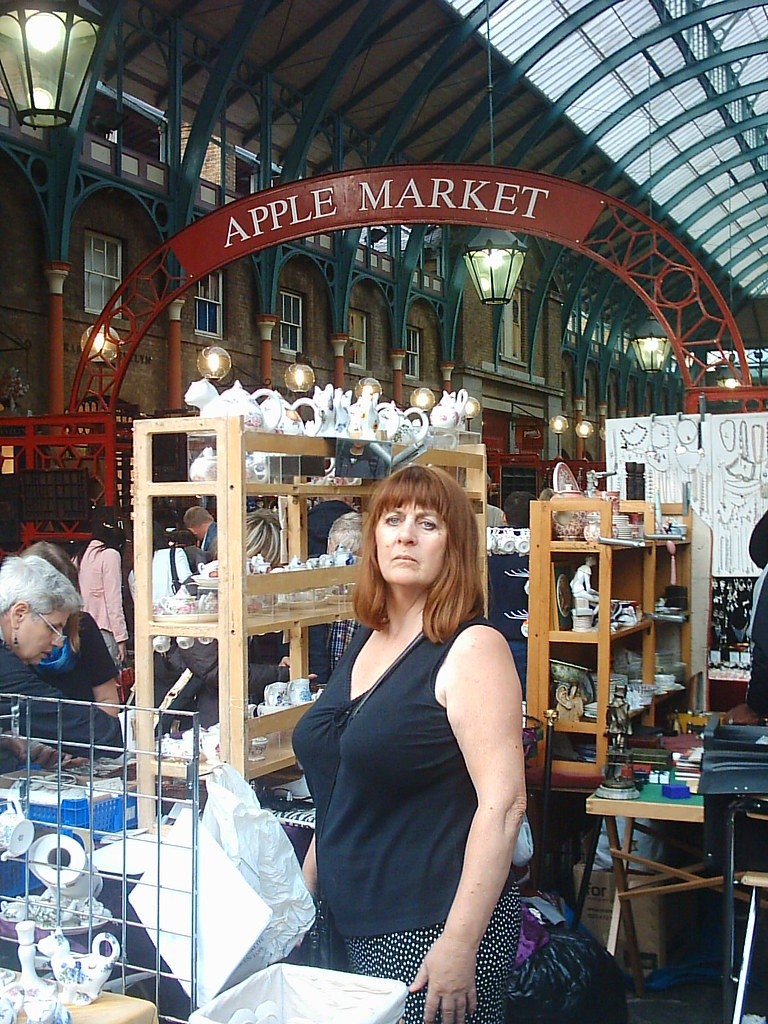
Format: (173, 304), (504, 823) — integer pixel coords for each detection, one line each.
(605, 683), (632, 753)
(0, 493), (364, 775)
(335, 440), (385, 480)
(746, 509), (768, 719)
(570, 553), (599, 614)
(292, 464), (526, 1024)
(484, 472), (572, 701)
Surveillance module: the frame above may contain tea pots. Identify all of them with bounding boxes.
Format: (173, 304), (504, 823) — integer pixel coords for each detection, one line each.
(0, 798), (35, 861)
(184, 379), (469, 450)
(252, 554), (264, 565)
(150, 586), (219, 614)
(285, 543), (357, 569)
(487, 527), (530, 556)
(198, 561), (219, 577)
(155, 723), (221, 764)
(152, 637), (213, 652)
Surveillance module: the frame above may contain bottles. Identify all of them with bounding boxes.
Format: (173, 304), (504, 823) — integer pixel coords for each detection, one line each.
(612, 524), (618, 539)
(550, 484), (587, 538)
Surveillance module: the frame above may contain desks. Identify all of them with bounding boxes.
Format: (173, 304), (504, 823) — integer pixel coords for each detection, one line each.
(0, 966), (160, 1024)
(0, 823), (340, 1015)
(587, 787), (742, 999)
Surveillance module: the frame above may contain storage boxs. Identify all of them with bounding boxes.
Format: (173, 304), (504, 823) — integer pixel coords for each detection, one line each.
(574, 860), (704, 970)
(0, 767), (144, 899)
(190, 963), (408, 1024)
(628, 732), (677, 777)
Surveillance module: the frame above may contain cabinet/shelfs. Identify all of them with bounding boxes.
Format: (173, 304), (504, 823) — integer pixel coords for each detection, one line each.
(131, 412), (494, 784)
(525, 494), (699, 784)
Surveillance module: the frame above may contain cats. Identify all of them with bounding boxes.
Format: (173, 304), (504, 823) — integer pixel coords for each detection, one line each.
(182, 375), (464, 441)
(37, 926), (121, 1007)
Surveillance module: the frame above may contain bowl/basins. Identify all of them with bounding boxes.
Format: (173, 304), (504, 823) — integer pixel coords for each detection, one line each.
(190, 457), (218, 482)
(629, 675), (675, 697)
(247, 736), (268, 761)
(550, 660), (591, 686)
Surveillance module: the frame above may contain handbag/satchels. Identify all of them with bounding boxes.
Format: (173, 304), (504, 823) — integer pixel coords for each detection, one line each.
(305, 900), (336, 971)
(151, 650), (200, 735)
(200, 764), (316, 995)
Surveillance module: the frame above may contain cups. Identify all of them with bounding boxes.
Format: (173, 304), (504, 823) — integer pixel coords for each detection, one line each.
(245, 562), (270, 576)
(248, 679), (325, 719)
(611, 600), (636, 627)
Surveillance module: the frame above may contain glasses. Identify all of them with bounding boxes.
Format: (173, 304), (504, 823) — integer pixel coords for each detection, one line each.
(37, 613), (68, 644)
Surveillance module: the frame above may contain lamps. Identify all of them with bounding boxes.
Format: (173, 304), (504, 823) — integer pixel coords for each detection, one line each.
(646, 413), (656, 456)
(0, 0), (105, 129)
(633, 50), (671, 373)
(718, 173), (748, 403)
(674, 412), (687, 455)
(464, 0), (528, 304)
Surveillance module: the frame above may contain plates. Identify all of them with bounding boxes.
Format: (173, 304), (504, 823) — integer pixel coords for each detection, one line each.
(326, 595), (354, 602)
(585, 702), (597, 718)
(587, 515), (633, 540)
(658, 684), (685, 695)
(191, 575), (219, 583)
(42, 909), (112, 935)
(153, 614), (219, 623)
(277, 597), (329, 609)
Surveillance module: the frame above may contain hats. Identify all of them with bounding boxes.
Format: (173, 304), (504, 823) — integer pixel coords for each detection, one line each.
(65, 538), (83, 554)
